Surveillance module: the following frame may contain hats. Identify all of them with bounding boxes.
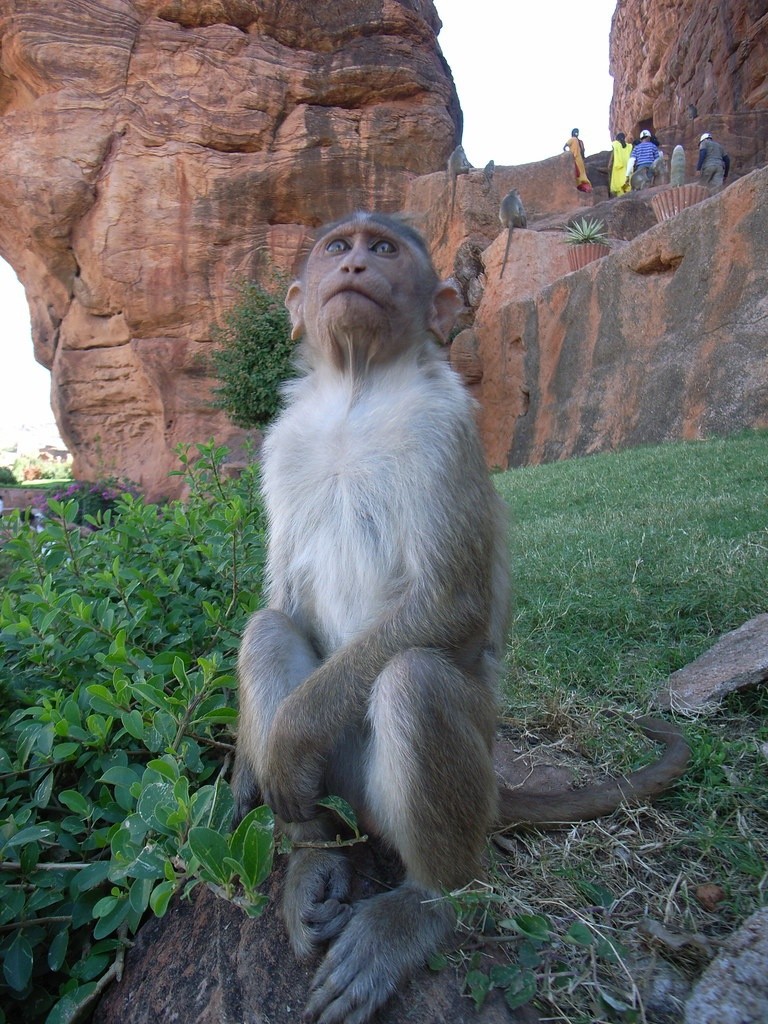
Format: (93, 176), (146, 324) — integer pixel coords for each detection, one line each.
(698, 133), (712, 146)
(640, 129), (651, 138)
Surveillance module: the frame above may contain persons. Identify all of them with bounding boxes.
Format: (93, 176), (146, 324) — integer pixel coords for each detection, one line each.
(696, 133), (730, 189)
(563, 129), (593, 193)
(607, 129), (664, 196)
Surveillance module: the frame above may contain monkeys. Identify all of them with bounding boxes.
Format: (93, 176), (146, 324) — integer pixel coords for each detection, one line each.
(499, 188), (527, 279)
(448, 145), (469, 209)
(630, 145), (687, 191)
(230, 210), (688, 1024)
